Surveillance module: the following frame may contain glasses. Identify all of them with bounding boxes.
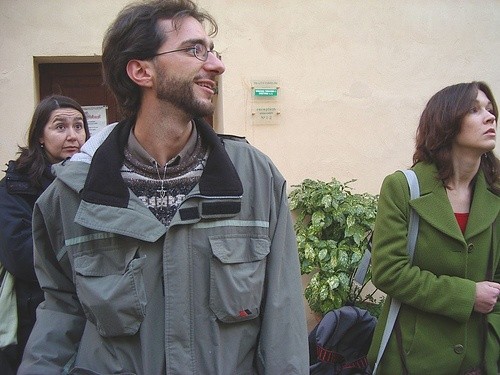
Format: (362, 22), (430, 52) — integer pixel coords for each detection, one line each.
(139, 43), (222, 62)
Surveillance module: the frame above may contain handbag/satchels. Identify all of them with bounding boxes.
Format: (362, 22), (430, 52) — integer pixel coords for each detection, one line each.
(308, 305), (377, 375)
(0, 264), (20, 351)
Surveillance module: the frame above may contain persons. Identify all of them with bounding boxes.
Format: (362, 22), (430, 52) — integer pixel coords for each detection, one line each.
(0, 94), (90, 375)
(15, 0), (311, 375)
(366, 81), (500, 375)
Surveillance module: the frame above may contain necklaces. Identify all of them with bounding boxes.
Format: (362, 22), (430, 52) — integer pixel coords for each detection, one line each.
(156, 162), (168, 200)
